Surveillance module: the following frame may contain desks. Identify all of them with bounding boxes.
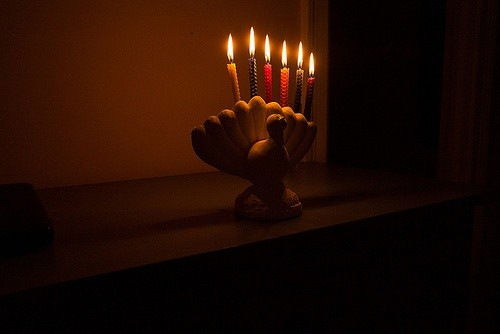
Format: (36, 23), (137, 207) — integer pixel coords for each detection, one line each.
(1, 162), (497, 334)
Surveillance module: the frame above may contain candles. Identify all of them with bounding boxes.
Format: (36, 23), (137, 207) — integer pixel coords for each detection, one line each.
(249, 27), (257, 101)
(264, 34), (273, 102)
(279, 40), (289, 107)
(226, 33), (240, 103)
(294, 41), (304, 114)
(304, 52), (315, 120)
